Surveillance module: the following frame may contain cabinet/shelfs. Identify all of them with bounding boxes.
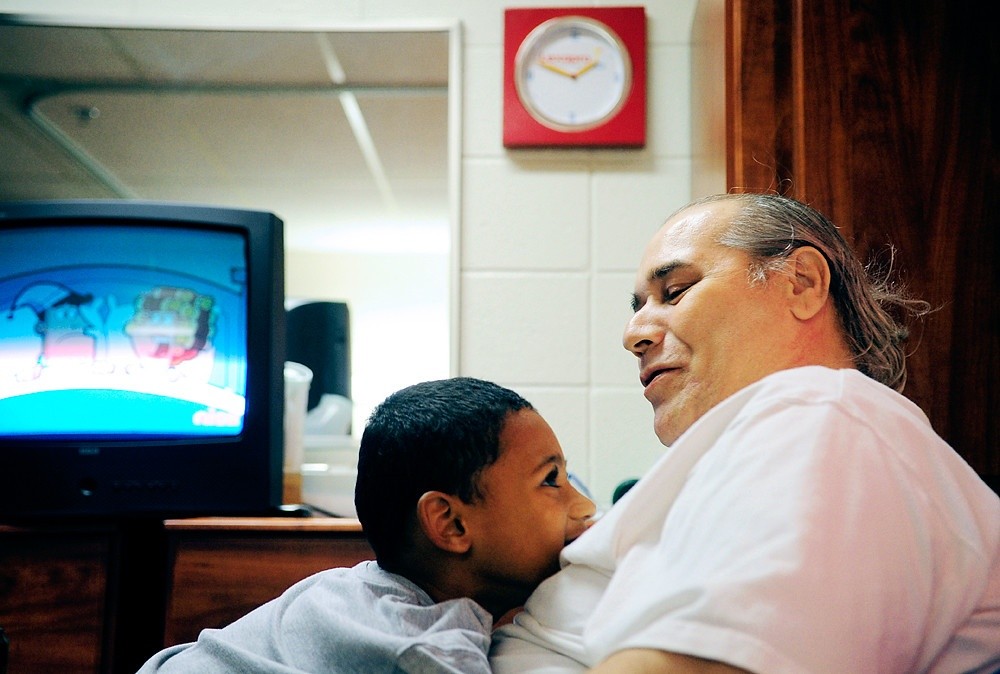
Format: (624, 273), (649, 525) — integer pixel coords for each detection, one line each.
(0, 511), (370, 674)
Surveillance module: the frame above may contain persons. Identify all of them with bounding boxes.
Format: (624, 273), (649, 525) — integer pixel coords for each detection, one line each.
(135, 377), (595, 674)
(487, 191), (1000, 674)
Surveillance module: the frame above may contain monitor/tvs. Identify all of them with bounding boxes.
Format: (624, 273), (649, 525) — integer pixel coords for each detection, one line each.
(0, 199), (288, 527)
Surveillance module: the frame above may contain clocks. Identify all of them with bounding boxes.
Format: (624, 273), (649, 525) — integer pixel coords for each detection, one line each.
(502, 6), (649, 147)
